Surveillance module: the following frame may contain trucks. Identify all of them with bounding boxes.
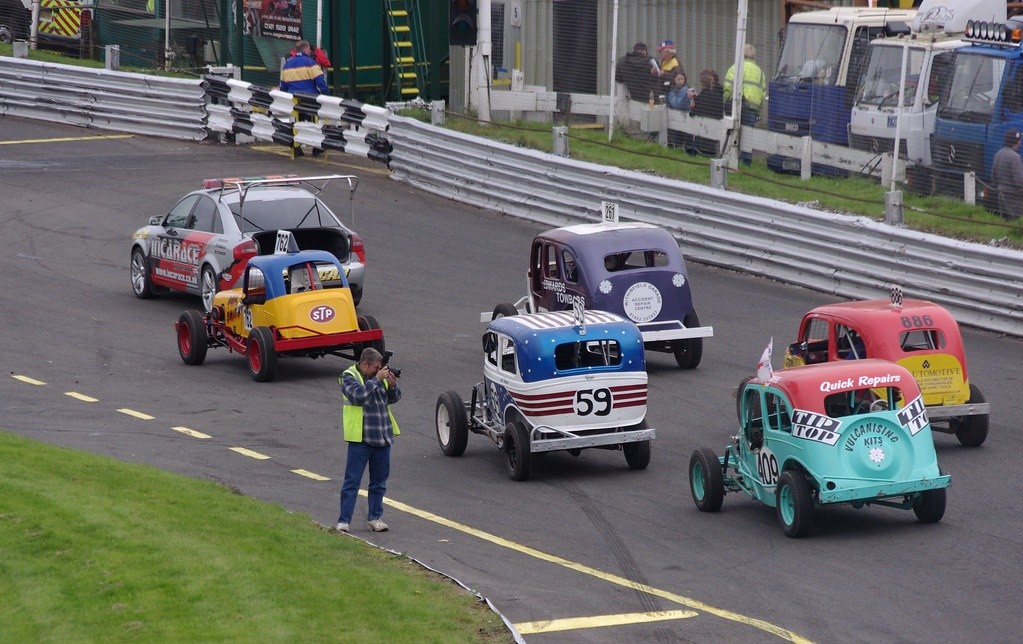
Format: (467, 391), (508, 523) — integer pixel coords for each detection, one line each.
(763, 5), (1022, 200)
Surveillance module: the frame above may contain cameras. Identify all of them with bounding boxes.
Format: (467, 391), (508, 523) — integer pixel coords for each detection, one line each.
(378, 350), (402, 379)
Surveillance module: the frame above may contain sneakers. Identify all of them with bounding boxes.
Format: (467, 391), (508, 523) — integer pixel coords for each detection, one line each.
(367, 520), (388, 532)
(338, 522), (350, 534)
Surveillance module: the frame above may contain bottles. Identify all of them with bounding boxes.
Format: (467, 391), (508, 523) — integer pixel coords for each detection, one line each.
(649, 90), (654, 109)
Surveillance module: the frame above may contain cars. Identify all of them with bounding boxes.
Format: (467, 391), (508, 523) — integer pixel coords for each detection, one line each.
(129, 173), (367, 314)
(476, 201), (712, 371)
(176, 230), (383, 382)
(786, 293), (989, 446)
(434, 295), (654, 481)
(690, 357), (950, 538)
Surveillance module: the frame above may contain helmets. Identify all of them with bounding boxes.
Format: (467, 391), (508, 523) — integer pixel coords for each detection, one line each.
(605, 256), (617, 270)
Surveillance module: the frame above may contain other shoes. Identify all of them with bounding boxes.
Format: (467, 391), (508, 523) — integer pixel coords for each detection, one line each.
(294, 147), (304, 157)
(312, 147), (325, 156)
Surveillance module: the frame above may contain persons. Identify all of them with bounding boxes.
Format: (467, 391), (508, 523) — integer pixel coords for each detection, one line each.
(616, 40), (723, 159)
(723, 44), (766, 165)
(280, 41), (330, 156)
(991, 129), (1023, 221)
(337, 348), (401, 533)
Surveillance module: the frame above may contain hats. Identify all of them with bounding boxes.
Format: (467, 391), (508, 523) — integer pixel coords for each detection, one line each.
(1005, 129), (1023, 146)
(658, 40), (675, 53)
